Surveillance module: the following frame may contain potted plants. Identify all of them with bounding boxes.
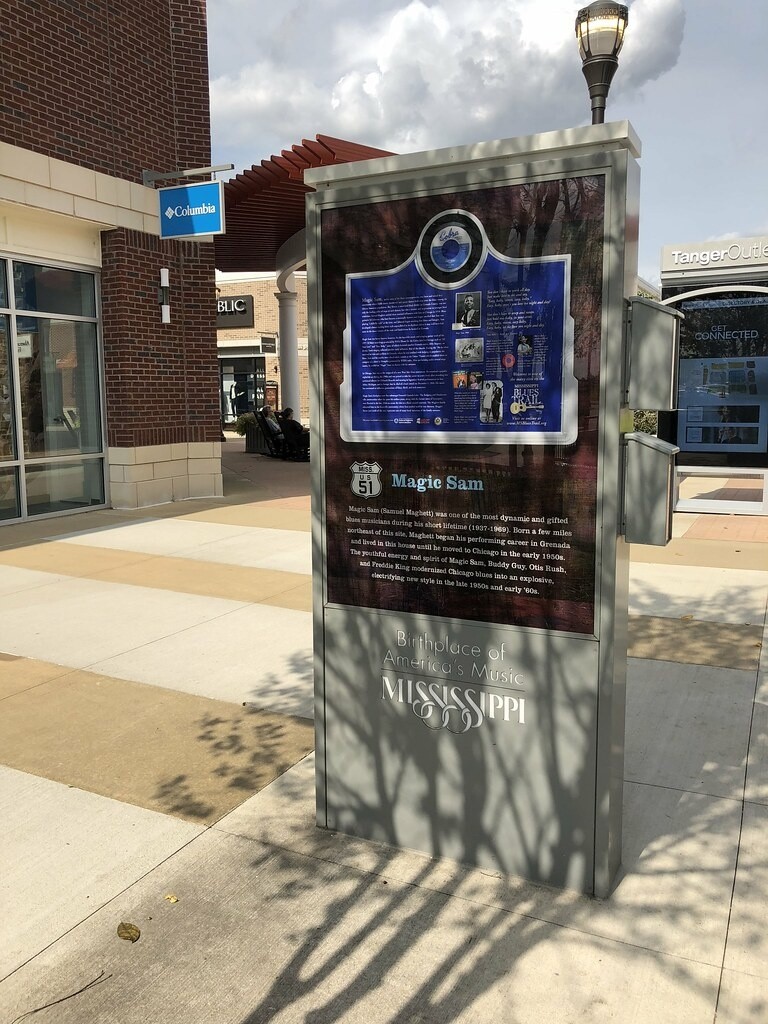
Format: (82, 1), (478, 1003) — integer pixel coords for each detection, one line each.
(234, 413), (270, 455)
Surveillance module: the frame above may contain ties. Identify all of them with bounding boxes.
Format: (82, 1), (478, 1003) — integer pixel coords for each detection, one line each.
(464, 311), (468, 323)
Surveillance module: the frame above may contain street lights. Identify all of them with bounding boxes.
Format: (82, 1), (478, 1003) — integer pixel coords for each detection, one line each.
(573, 1), (631, 124)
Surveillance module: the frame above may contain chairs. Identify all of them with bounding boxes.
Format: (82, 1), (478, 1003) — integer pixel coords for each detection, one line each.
(252, 410), (291, 459)
(273, 411), (311, 462)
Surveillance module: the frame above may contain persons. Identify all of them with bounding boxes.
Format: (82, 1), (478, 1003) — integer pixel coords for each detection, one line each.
(718, 406), (741, 423)
(517, 336), (532, 357)
(718, 427), (742, 444)
(482, 382), (502, 422)
(457, 295), (481, 327)
(283, 408), (310, 448)
(260, 405), (284, 441)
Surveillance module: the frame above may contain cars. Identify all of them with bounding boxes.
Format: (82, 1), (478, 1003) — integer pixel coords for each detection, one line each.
(2, 406), (81, 457)
(678, 384), (729, 412)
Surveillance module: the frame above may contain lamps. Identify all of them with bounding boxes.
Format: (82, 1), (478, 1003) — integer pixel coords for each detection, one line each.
(159, 268), (169, 288)
(160, 305), (171, 323)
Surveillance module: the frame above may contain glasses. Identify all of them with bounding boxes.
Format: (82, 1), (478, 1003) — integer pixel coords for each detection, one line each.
(269, 411), (273, 413)
